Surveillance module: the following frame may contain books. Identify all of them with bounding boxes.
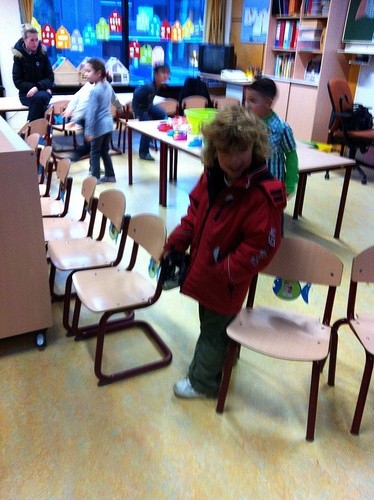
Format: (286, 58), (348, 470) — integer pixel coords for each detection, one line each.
(273, 0), (331, 81)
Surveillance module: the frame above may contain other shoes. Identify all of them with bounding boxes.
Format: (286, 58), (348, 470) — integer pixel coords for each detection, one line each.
(173, 375), (207, 399)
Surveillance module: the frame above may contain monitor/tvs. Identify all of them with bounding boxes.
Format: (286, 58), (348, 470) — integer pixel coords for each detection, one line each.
(197, 44), (239, 74)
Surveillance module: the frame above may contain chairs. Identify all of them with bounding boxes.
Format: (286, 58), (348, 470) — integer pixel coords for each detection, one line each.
(15, 95), (374, 442)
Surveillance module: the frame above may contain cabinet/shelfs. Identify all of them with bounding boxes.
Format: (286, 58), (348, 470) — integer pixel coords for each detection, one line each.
(262, 0), (366, 145)
(0, 115), (54, 349)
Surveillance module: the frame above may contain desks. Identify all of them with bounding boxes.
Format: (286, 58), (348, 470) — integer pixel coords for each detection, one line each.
(124, 119), (356, 240)
(199, 72), (255, 107)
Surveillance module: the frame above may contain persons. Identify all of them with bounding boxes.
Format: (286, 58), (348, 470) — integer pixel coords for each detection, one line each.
(160, 105), (288, 398)
(132, 63), (171, 160)
(61, 58), (124, 184)
(244, 77), (300, 240)
(10, 26), (56, 122)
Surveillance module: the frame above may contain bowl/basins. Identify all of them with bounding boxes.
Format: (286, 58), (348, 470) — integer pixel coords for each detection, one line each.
(184, 108), (221, 133)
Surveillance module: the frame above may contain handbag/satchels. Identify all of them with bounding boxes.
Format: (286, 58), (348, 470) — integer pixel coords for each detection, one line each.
(339, 94), (373, 131)
